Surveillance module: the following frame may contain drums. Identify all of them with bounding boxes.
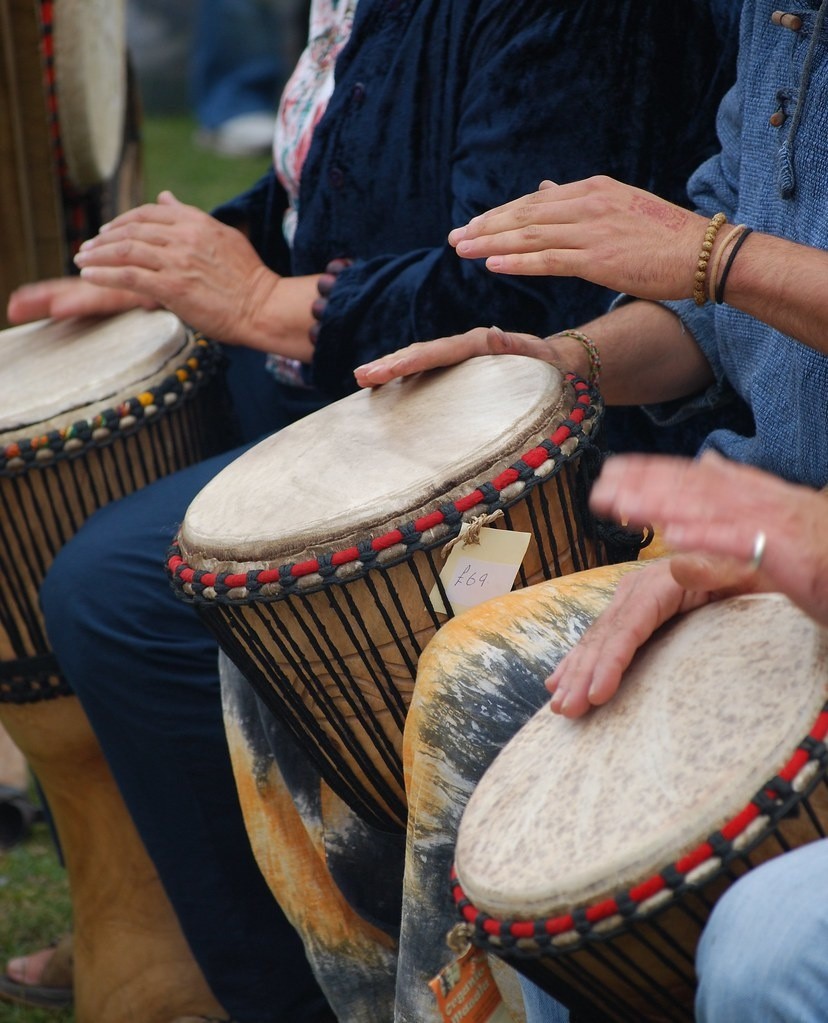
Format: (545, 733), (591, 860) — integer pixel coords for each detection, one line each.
(165, 346), (611, 834)
(0, 0), (132, 300)
(452, 579), (828, 1023)
(1, 302), (273, 1023)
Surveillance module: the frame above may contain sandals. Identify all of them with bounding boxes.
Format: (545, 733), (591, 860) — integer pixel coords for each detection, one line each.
(1, 936), (75, 1011)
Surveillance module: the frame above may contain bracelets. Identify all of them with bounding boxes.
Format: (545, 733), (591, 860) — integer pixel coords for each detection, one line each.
(694, 210), (754, 307)
(308, 257), (364, 359)
(545, 330), (604, 390)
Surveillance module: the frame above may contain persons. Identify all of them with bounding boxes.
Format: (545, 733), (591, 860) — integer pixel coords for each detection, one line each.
(7, 0), (725, 1023)
(543, 448), (828, 1023)
(214, 0), (828, 1023)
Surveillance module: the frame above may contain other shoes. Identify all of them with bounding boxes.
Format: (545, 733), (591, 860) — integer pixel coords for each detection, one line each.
(191, 109), (281, 160)
(0, 789), (36, 853)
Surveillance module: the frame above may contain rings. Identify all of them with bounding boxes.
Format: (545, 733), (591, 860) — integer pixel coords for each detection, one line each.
(749, 530), (769, 572)
